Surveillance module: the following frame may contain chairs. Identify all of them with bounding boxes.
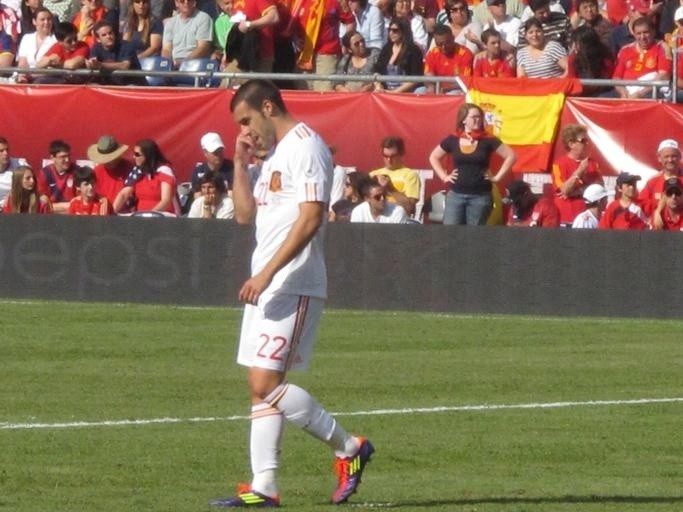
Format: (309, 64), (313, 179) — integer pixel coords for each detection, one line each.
(134, 55), (173, 87)
(173, 58), (222, 90)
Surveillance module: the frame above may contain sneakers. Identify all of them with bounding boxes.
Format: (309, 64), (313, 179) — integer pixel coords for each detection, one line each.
(209, 483), (279, 508)
(331, 436), (375, 505)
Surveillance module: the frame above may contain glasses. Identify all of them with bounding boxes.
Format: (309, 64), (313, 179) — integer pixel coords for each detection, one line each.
(133, 151), (143, 157)
(371, 192), (387, 201)
(387, 27), (400, 33)
(435, 39), (453, 46)
(451, 4), (466, 12)
(354, 38), (364, 47)
(65, 35), (78, 45)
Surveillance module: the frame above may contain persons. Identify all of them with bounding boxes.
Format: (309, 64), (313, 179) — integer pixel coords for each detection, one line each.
(214, 79), (374, 507)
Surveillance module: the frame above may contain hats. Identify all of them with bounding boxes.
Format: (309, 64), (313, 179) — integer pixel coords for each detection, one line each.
(87, 134), (129, 163)
(200, 132), (225, 153)
(583, 139), (683, 204)
